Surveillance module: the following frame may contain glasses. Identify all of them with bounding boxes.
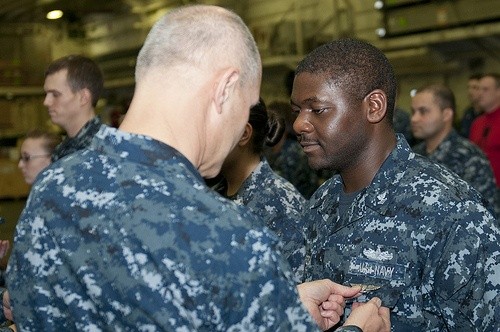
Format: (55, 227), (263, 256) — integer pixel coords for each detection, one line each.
(18, 152), (50, 162)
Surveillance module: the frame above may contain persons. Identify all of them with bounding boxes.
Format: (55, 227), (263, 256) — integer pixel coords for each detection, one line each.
(41, 54), (106, 167)
(0, 74), (500, 332)
(6, 6), (391, 332)
(283, 38), (500, 332)
